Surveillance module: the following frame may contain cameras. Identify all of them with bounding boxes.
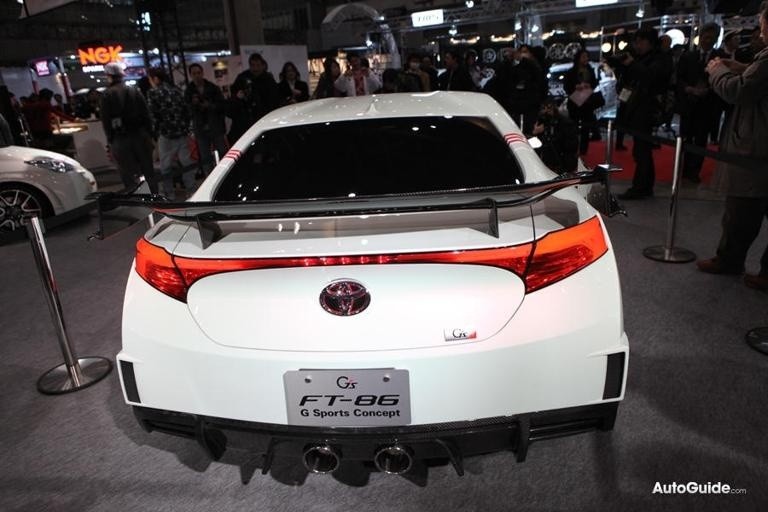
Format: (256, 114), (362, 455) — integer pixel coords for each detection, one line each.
(536, 113), (552, 127)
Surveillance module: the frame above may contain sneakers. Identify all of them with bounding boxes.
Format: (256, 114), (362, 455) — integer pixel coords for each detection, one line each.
(122, 191), (196, 201)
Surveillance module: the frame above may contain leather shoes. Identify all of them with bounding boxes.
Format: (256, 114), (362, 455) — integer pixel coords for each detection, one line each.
(615, 187), (646, 200)
(743, 273), (768, 292)
(645, 187), (654, 196)
(695, 257), (745, 274)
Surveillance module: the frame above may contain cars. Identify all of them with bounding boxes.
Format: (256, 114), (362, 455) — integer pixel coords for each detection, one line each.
(0, 142), (103, 237)
(541, 57), (619, 114)
(104, 83), (636, 476)
(119, 62), (146, 86)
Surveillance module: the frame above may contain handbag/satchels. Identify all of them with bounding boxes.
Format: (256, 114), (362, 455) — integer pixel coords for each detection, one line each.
(649, 89), (677, 127)
